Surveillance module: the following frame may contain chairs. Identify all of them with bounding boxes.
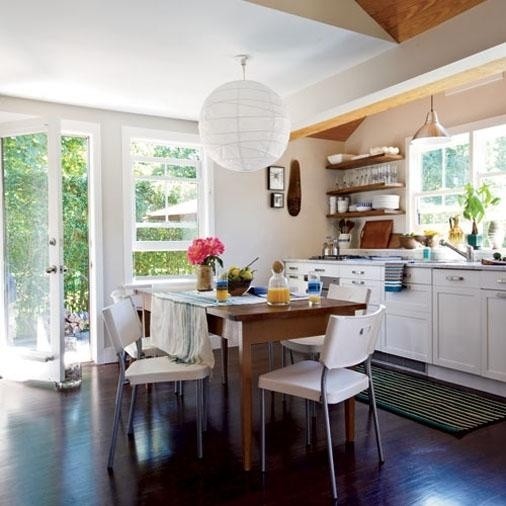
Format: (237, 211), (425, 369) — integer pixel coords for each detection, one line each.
(96, 298), (210, 473)
(281, 277), (371, 418)
(110, 287), (187, 397)
(258, 304), (386, 500)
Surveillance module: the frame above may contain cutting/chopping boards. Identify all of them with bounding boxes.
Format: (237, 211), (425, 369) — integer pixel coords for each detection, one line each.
(359, 220), (393, 249)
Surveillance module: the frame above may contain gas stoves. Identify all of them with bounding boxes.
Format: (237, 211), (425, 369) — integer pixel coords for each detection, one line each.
(308, 254), (370, 261)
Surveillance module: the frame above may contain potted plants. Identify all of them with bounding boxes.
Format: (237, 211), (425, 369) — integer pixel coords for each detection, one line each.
(454, 179), (502, 250)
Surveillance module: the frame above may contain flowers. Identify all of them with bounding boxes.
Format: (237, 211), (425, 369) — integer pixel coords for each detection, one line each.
(186, 236), (225, 268)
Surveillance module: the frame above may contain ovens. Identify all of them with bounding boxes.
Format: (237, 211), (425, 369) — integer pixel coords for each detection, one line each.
(305, 274), (340, 299)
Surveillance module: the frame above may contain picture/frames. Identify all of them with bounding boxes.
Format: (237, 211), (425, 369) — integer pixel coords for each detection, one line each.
(265, 165), (287, 189)
(270, 190), (285, 208)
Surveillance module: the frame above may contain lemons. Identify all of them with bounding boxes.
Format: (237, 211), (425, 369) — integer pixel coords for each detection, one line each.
(422, 228), (434, 236)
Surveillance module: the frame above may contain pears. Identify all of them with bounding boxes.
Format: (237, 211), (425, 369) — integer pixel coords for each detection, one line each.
(221, 266), (253, 281)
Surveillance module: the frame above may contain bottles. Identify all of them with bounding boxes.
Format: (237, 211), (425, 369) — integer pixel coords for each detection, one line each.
(307, 275), (321, 305)
(322, 239), (338, 257)
(215, 280), (229, 302)
(267, 261), (291, 306)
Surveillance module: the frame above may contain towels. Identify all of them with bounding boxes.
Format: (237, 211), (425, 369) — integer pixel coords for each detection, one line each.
(384, 259), (408, 291)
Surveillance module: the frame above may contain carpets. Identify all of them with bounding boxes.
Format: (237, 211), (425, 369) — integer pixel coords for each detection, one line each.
(346, 363), (506, 437)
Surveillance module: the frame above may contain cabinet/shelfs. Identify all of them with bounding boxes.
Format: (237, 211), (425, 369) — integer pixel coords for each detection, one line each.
(340, 265), (383, 353)
(325, 153), (406, 217)
(435, 262), (506, 383)
(382, 262), (434, 365)
(285, 257), (340, 293)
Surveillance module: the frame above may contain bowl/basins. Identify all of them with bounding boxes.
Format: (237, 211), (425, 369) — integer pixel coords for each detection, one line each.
(228, 279), (252, 296)
(417, 235), (438, 247)
(328, 154), (354, 165)
(370, 147), (400, 155)
(399, 235), (419, 249)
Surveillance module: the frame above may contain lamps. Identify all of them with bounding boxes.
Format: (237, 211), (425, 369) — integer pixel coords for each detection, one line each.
(411, 93), (451, 145)
(197, 55), (292, 173)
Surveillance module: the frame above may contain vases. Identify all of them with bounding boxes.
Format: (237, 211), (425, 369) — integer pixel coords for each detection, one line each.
(195, 265), (214, 291)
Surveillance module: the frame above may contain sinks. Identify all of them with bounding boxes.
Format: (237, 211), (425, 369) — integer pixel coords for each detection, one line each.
(413, 260), (463, 263)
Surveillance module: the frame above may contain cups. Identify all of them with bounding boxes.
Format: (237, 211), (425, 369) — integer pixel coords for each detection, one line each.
(335, 165), (399, 190)
(349, 205), (356, 212)
(338, 233), (352, 249)
(468, 234), (481, 249)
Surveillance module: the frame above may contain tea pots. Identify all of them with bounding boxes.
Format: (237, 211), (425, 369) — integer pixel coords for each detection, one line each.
(448, 216), (465, 245)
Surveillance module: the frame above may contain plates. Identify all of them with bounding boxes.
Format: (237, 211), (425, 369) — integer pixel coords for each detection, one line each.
(356, 206), (372, 212)
(371, 194), (401, 209)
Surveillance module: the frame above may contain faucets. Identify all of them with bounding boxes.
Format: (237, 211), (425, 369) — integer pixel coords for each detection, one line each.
(440, 239), (474, 262)
